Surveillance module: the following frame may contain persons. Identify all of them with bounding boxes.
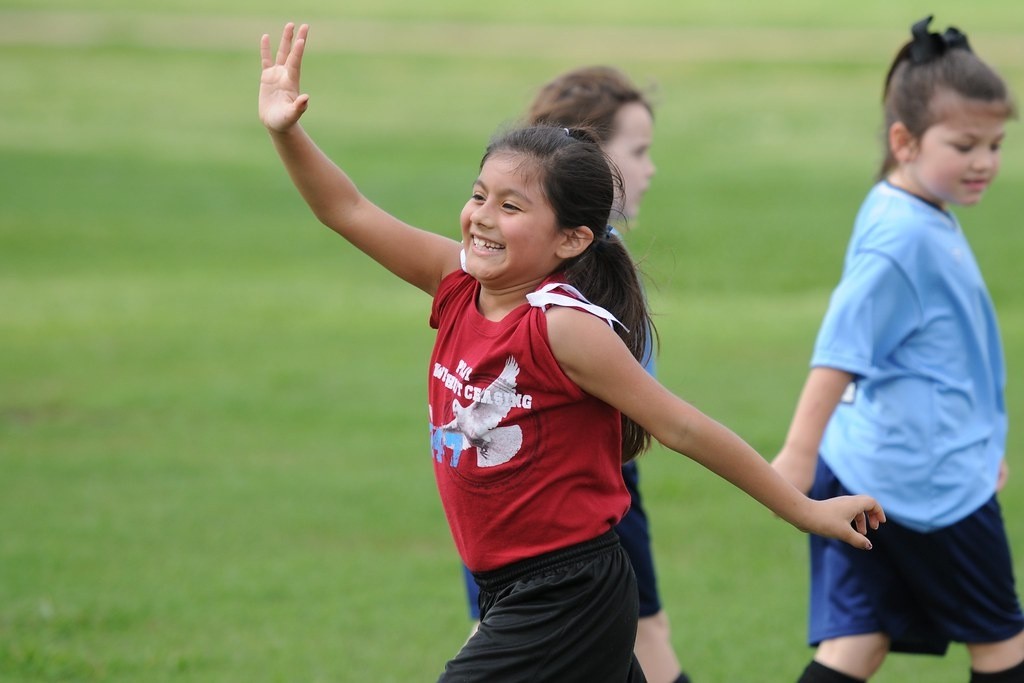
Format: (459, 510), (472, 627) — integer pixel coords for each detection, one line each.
(462, 66), (695, 683)
(258, 21), (885, 683)
(769, 14), (1023, 683)
